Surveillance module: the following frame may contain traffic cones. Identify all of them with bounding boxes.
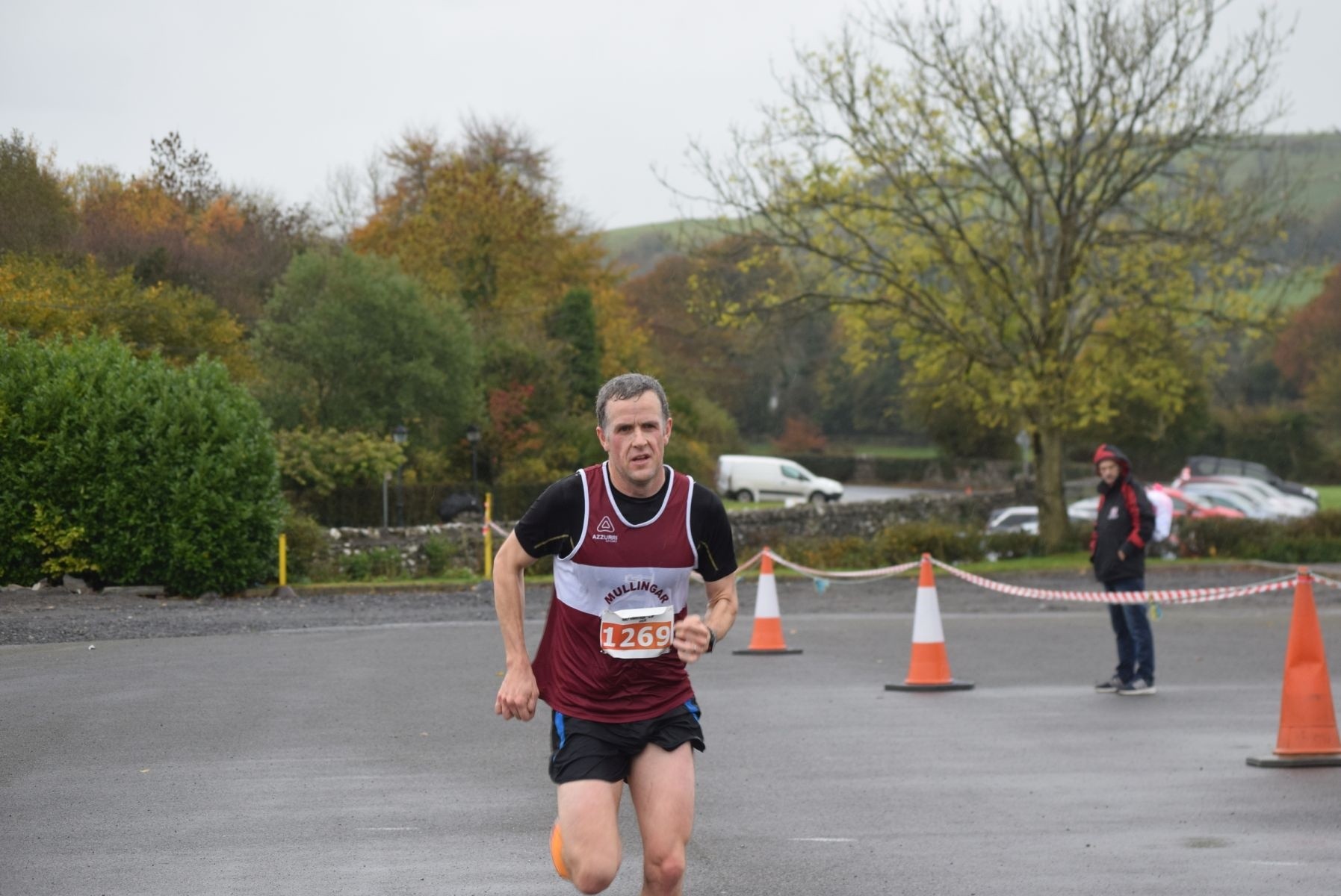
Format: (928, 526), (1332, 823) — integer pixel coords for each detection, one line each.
(884, 552), (976, 692)
(731, 545), (804, 656)
(1245, 566), (1341, 769)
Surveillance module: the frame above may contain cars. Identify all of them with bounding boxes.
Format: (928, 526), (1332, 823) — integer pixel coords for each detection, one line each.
(982, 453), (1322, 561)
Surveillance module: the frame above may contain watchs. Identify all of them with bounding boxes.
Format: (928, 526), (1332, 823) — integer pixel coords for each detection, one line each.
(706, 627), (717, 654)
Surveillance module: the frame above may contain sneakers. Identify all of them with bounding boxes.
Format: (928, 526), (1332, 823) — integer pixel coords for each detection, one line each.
(1097, 675), (1124, 692)
(1118, 675), (1156, 695)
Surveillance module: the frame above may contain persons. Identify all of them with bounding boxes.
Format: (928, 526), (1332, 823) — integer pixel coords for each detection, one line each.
(1178, 466), (1191, 479)
(1089, 443), (1157, 695)
(492, 374), (738, 896)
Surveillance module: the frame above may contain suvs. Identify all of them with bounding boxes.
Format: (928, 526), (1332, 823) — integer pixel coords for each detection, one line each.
(715, 453), (845, 508)
